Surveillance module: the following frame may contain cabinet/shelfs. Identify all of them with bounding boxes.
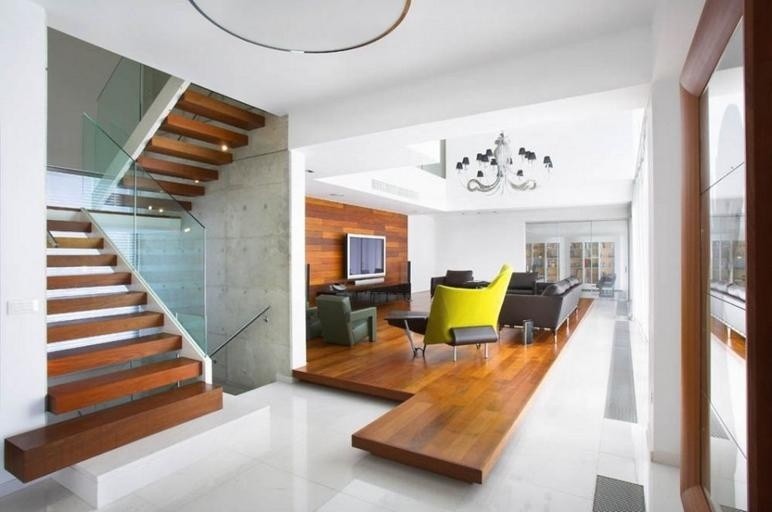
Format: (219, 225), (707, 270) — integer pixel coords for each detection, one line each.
(316, 281), (410, 307)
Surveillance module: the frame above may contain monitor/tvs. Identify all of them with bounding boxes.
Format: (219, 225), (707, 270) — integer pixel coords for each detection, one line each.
(345, 232), (387, 281)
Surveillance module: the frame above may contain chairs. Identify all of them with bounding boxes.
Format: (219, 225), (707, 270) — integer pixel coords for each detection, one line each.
(306, 300), (323, 340)
(383, 263), (513, 363)
(315, 294), (378, 347)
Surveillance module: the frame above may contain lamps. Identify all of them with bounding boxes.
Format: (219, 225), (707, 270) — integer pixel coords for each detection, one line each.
(456, 131), (554, 196)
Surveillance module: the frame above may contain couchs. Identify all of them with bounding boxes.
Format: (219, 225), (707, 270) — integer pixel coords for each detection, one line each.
(430, 270), (584, 344)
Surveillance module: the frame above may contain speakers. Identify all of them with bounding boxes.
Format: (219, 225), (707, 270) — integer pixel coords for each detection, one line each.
(354, 277), (385, 286)
